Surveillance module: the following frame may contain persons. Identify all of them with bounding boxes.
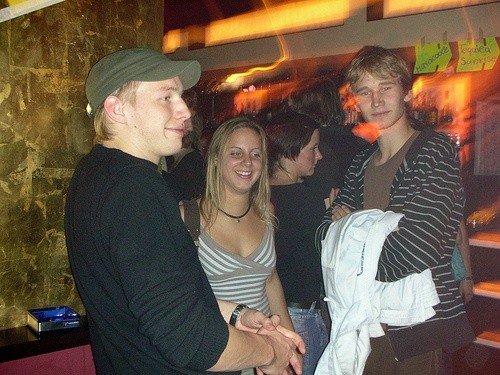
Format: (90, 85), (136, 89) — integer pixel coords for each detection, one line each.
(63, 46), (473, 375)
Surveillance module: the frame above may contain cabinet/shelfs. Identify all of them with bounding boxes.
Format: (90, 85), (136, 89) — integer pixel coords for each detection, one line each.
(467, 212), (500, 349)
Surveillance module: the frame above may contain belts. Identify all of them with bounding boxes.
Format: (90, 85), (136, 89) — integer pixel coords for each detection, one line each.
(287, 302), (321, 309)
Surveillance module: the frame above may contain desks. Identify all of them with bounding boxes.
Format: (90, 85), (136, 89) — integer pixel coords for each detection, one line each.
(0, 315), (95, 375)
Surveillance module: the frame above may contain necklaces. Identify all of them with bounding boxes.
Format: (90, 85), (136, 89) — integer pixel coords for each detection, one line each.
(211, 200), (252, 222)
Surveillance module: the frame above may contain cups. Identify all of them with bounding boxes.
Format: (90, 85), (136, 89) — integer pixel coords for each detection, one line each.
(448, 133), (462, 147)
(469, 219), (481, 232)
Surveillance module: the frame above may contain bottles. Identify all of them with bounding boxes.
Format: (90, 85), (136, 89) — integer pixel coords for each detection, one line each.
(419, 96), (438, 127)
(240, 101), (256, 116)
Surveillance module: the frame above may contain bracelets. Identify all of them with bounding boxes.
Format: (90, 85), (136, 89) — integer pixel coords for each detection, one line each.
(463, 277), (473, 280)
(229, 304), (249, 327)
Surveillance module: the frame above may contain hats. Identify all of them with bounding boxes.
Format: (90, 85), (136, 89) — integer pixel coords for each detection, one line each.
(85, 48), (201, 118)
